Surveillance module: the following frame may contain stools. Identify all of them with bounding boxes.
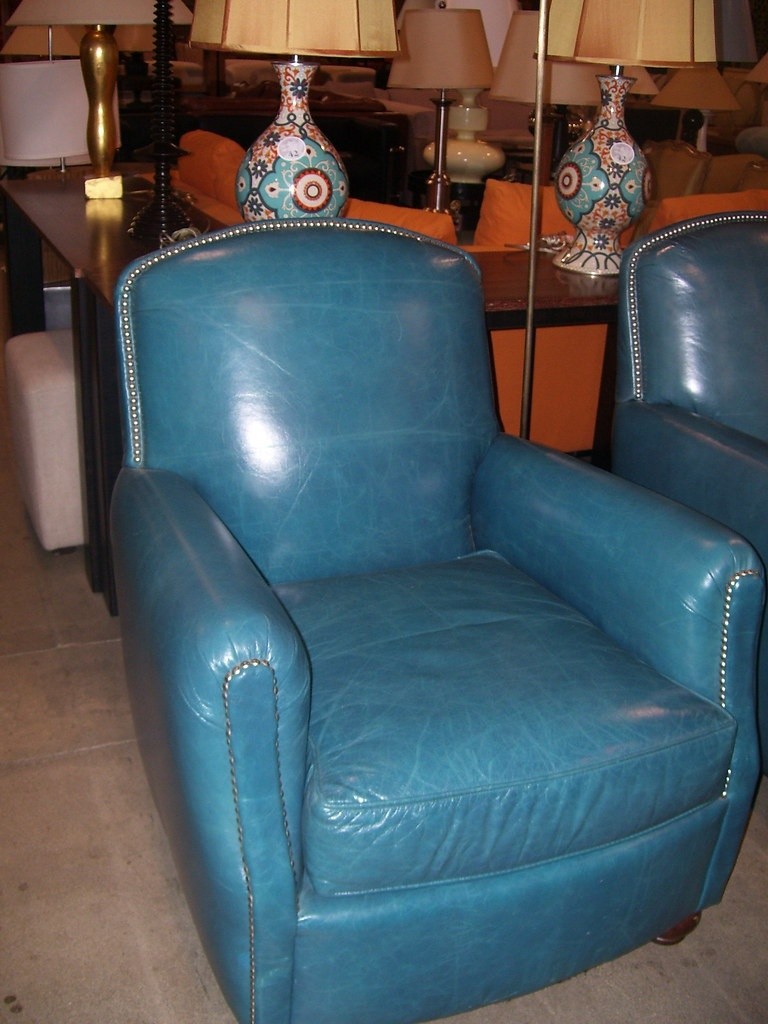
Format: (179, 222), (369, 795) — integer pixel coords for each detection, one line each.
(5, 329), (85, 557)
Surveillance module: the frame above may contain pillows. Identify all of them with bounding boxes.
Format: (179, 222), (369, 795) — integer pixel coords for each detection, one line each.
(345, 197), (459, 247)
(176, 128), (248, 214)
(472, 178), (640, 250)
(646, 188), (768, 235)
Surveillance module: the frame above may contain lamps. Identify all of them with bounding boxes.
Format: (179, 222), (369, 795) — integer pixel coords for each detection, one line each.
(490, 10), (615, 172)
(187, 0), (401, 223)
(533, 0), (719, 276)
(0, 59), (121, 169)
(651, 64), (740, 152)
(396, 0), (522, 184)
(743, 53), (768, 88)
(621, 67), (661, 96)
(386, 1), (495, 233)
(7, 0), (195, 198)
(0, 25), (156, 57)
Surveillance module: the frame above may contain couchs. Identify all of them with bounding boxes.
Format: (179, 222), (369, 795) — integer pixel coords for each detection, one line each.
(611, 212), (768, 777)
(0, 57), (768, 618)
(109, 217), (768, 1024)
